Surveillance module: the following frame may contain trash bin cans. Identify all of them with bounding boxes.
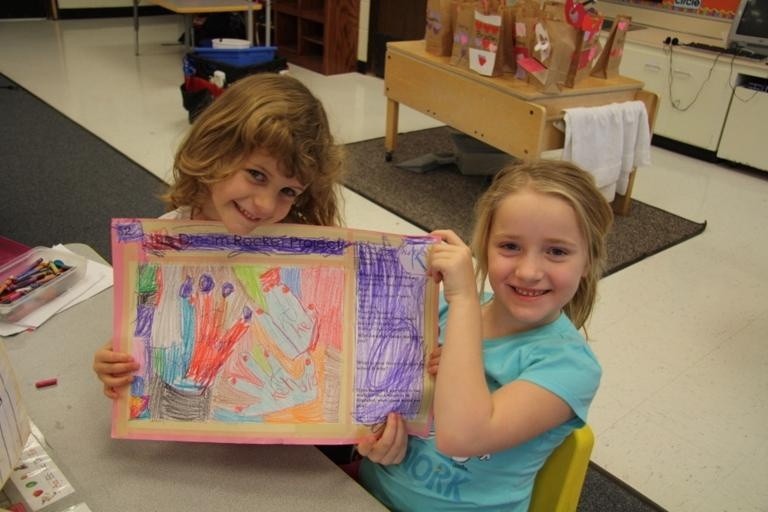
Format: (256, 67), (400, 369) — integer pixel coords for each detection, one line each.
(183, 47), (279, 125)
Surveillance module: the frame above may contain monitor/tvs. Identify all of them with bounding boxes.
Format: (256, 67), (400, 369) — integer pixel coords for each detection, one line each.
(725, 0), (768, 57)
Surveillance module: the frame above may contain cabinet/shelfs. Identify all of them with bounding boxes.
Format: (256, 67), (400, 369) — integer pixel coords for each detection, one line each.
(717, 60), (768, 178)
(583, 27), (738, 163)
(272, 1), (359, 76)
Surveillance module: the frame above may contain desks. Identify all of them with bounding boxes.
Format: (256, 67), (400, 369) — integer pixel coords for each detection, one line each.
(1, 243), (393, 512)
(384, 39), (662, 217)
(132, 0), (263, 55)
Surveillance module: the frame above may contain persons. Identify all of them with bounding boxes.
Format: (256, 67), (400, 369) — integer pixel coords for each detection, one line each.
(338, 158), (615, 510)
(94, 73), (345, 400)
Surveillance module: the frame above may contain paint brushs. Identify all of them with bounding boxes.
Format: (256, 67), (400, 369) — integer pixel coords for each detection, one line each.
(0, 257), (72, 322)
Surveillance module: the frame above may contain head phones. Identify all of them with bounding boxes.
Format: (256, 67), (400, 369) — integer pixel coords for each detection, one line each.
(665, 33), (680, 46)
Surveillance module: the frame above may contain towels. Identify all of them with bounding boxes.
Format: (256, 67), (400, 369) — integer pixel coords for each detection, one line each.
(564, 100), (652, 204)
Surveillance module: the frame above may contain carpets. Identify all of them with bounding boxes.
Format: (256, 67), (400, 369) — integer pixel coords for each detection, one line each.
(334, 125), (706, 283)
(0, 73), (666, 511)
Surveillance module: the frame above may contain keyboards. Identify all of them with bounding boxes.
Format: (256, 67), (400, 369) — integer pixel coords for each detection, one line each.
(686, 41), (768, 62)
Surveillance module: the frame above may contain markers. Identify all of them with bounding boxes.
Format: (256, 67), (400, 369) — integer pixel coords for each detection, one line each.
(35, 378), (56, 388)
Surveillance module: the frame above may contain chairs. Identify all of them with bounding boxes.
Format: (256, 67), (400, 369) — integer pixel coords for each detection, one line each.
(191, 45), (278, 71)
(528, 424), (594, 512)
